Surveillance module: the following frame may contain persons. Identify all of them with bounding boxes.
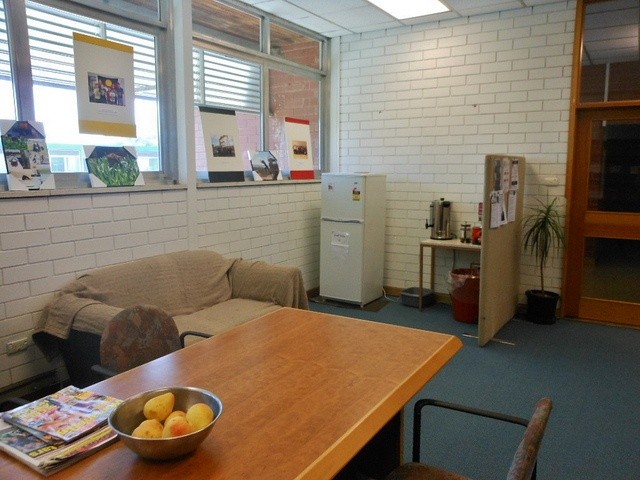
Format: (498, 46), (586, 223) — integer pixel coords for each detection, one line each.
(91, 79), (124, 105)
(217, 134), (232, 156)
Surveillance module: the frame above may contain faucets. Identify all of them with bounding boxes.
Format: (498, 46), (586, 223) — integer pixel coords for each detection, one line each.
(425, 219), (433, 229)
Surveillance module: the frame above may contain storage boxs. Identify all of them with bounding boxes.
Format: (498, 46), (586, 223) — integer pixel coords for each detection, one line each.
(400, 286), (433, 307)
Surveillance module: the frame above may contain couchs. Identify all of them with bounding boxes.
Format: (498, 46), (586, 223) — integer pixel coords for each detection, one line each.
(34, 248), (309, 377)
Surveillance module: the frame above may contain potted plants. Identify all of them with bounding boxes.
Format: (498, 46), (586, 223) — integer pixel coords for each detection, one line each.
(521, 192), (566, 323)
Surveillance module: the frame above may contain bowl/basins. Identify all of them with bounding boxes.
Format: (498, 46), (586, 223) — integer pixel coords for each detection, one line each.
(107, 386), (223, 461)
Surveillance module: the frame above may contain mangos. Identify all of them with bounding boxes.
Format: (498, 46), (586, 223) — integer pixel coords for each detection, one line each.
(164, 409), (184, 422)
(186, 402), (213, 429)
(160, 415), (190, 438)
(143, 392), (175, 421)
(131, 419), (162, 436)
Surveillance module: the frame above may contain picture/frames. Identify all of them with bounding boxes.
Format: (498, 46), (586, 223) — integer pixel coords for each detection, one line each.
(0, 116), (57, 189)
(81, 141), (143, 188)
(247, 148), (283, 181)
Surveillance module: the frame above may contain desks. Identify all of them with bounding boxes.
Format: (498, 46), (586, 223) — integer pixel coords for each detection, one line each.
(418, 238), (482, 314)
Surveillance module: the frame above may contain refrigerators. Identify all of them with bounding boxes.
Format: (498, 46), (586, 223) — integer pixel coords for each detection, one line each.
(319, 173), (386, 308)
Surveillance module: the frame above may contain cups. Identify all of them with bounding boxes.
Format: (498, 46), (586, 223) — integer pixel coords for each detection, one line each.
(460, 221), (472, 244)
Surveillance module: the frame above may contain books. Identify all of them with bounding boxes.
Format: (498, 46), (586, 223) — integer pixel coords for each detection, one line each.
(0, 385), (122, 477)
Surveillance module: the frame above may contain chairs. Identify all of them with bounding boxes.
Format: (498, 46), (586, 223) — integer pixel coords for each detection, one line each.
(403, 395), (554, 480)
